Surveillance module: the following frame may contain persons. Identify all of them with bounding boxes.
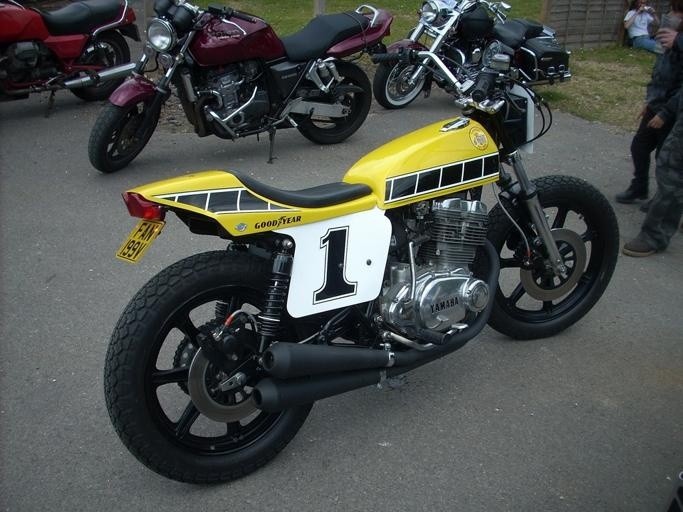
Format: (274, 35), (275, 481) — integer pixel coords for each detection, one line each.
(622, 0), (664, 55)
(621, 26), (683, 260)
(612, 21), (683, 205)
(659, 1), (683, 33)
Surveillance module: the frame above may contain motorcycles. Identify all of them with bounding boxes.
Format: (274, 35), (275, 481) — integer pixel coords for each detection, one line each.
(89, 1), (395, 174)
(105, 16), (620, 484)
(373, 0), (573, 110)
(0, 0), (141, 120)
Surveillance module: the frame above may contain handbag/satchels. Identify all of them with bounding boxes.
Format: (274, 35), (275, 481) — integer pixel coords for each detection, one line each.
(647, 21), (660, 37)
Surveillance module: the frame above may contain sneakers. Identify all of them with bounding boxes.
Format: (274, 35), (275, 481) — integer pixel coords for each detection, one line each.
(622, 233), (666, 257)
(639, 199), (652, 211)
(616, 186), (649, 204)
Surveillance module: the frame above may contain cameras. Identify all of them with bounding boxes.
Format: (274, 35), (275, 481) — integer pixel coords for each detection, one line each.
(644, 6), (651, 11)
